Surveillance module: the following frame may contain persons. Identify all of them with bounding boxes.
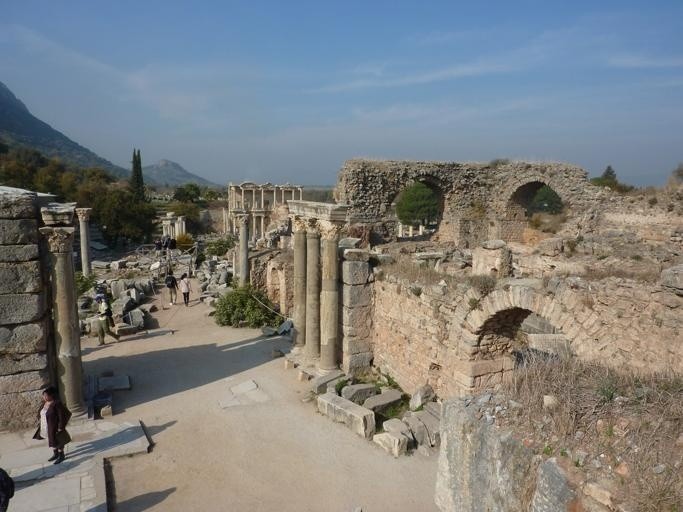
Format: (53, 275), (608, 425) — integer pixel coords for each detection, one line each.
(95, 295), (120, 346)
(153, 235), (176, 258)
(165, 270), (178, 306)
(32, 388), (71, 465)
(179, 273), (192, 308)
(194, 252), (206, 270)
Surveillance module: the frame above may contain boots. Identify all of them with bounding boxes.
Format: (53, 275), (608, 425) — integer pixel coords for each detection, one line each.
(48, 449), (64, 464)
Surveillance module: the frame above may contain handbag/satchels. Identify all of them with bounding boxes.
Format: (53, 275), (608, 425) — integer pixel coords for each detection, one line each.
(55, 407), (72, 427)
(55, 430), (71, 447)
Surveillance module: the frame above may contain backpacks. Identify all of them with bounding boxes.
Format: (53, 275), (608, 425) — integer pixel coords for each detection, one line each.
(167, 280), (174, 287)
